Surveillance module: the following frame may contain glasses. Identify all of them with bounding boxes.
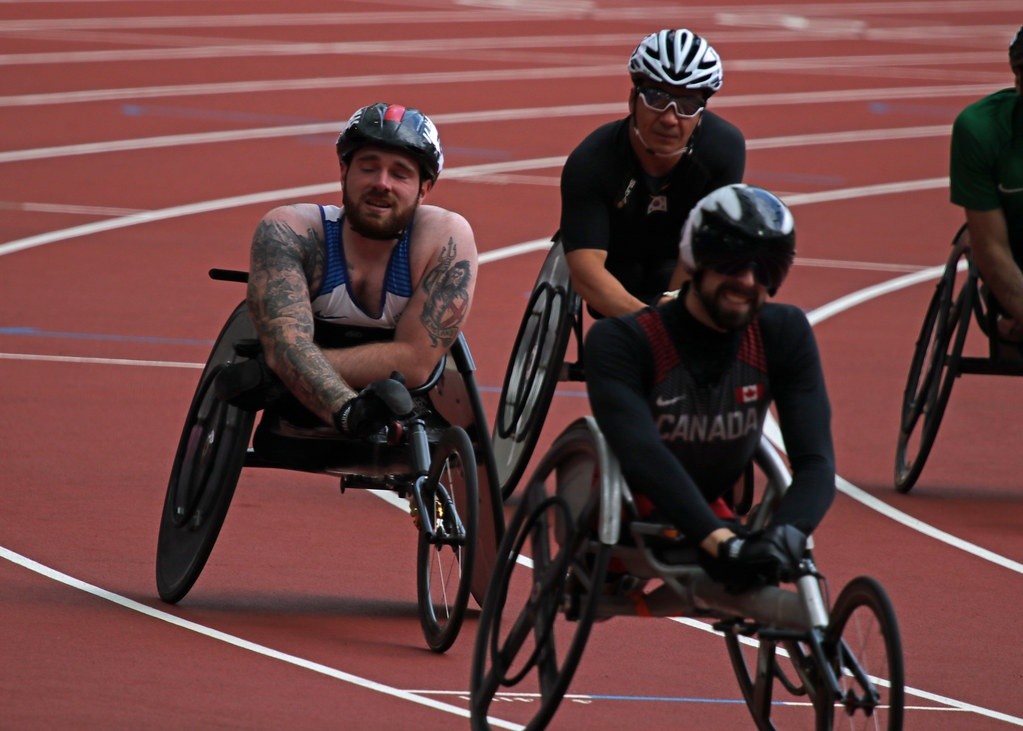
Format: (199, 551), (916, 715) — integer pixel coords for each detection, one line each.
(639, 87), (705, 118)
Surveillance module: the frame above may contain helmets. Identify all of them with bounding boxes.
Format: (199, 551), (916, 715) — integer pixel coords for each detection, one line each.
(678, 183), (796, 298)
(1009, 26), (1023, 67)
(627, 28), (723, 91)
(335, 102), (444, 186)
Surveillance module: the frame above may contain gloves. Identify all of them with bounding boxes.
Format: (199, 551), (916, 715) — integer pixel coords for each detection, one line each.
(214, 338), (285, 413)
(333, 378), (416, 444)
(716, 524), (807, 594)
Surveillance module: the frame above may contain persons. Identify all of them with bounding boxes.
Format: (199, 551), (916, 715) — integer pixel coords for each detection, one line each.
(558, 28), (746, 322)
(583, 185), (835, 593)
(949, 24), (1023, 322)
(215, 101), (479, 446)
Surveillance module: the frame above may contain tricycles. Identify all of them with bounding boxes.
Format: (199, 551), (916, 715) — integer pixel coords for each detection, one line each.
(155, 269), (510, 652)
(468, 417), (906, 731)
(488, 228), (787, 515)
(892, 223), (1023, 493)
